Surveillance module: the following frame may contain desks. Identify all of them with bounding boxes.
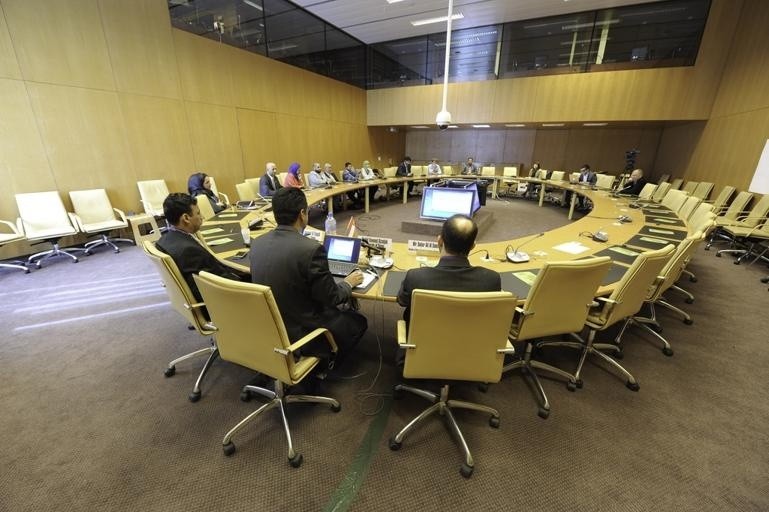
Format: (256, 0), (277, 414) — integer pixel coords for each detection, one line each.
(501, 176), (681, 220)
(201, 184), (382, 303)
(327, 174), (500, 221)
(381, 217), (690, 305)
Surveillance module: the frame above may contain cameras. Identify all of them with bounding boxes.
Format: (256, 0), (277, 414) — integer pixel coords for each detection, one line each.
(624, 149), (641, 165)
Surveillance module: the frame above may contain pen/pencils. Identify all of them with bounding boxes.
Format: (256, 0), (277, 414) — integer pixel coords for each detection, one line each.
(366, 269), (377, 276)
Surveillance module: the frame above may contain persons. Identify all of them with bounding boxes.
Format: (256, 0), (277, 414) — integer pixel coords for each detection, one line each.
(323, 163), (338, 182)
(578, 164), (597, 208)
(284, 162), (304, 188)
(395, 215), (502, 346)
(187, 173), (230, 213)
(620, 168), (645, 195)
(343, 162), (361, 204)
(259, 162), (283, 197)
(461, 157), (478, 179)
(154, 192), (238, 322)
(249, 186), (369, 358)
(521, 161), (542, 198)
(395, 157), (414, 199)
(427, 158), (441, 186)
(308, 163), (332, 186)
(361, 160), (378, 202)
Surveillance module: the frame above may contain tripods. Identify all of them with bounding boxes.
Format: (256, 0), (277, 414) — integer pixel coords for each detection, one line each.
(614, 164), (634, 190)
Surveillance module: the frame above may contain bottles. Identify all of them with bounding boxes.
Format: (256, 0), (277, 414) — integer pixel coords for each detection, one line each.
(324, 211), (337, 236)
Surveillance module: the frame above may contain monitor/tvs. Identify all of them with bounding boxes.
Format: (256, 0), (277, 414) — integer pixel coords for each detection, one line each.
(430, 181), (446, 187)
(448, 179), (488, 206)
(464, 180), (481, 213)
(420, 187), (475, 220)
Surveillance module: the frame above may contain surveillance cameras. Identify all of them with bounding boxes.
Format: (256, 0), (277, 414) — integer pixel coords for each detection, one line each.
(436, 112), (452, 129)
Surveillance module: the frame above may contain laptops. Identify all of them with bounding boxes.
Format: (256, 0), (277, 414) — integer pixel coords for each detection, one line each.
(568, 174), (580, 184)
(323, 235), (361, 277)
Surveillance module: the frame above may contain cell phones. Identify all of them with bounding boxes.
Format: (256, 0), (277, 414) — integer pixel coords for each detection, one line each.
(234, 251), (247, 258)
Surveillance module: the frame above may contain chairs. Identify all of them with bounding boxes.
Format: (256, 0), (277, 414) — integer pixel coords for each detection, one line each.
(244, 178), (261, 201)
(661, 189), (675, 206)
(704, 194), (768, 251)
(653, 182), (671, 202)
(692, 181), (714, 203)
(209, 176), (231, 205)
(383, 168), (395, 177)
(411, 166), (424, 182)
(280, 172), (287, 185)
(704, 191), (753, 251)
(688, 203), (715, 226)
(481, 166), (495, 176)
(422, 166), (429, 182)
(639, 183), (658, 200)
(570, 172), (581, 179)
(682, 181), (699, 196)
(69, 188), (134, 256)
(14, 190), (96, 270)
(715, 216), (769, 267)
(503, 167), (517, 177)
(696, 219), (718, 236)
(477, 255), (612, 420)
(704, 211), (717, 221)
(235, 183), (252, 201)
(550, 171), (565, 180)
(136, 179), (173, 234)
(595, 174), (616, 190)
(673, 193), (688, 213)
(141, 240), (238, 403)
(701, 185), (735, 217)
(304, 173), (310, 186)
(612, 231), (707, 356)
(195, 194), (215, 220)
(190, 270), (341, 469)
(388, 288), (517, 479)
(441, 166), (452, 179)
(671, 178), (683, 190)
(533, 243), (677, 392)
(392, 167), (398, 174)
(541, 170), (548, 180)
(339, 171), (344, 182)
(678, 196), (702, 223)
(0, 219), (30, 276)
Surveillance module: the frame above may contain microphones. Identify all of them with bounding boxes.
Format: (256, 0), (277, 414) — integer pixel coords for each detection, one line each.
(507, 233), (544, 263)
